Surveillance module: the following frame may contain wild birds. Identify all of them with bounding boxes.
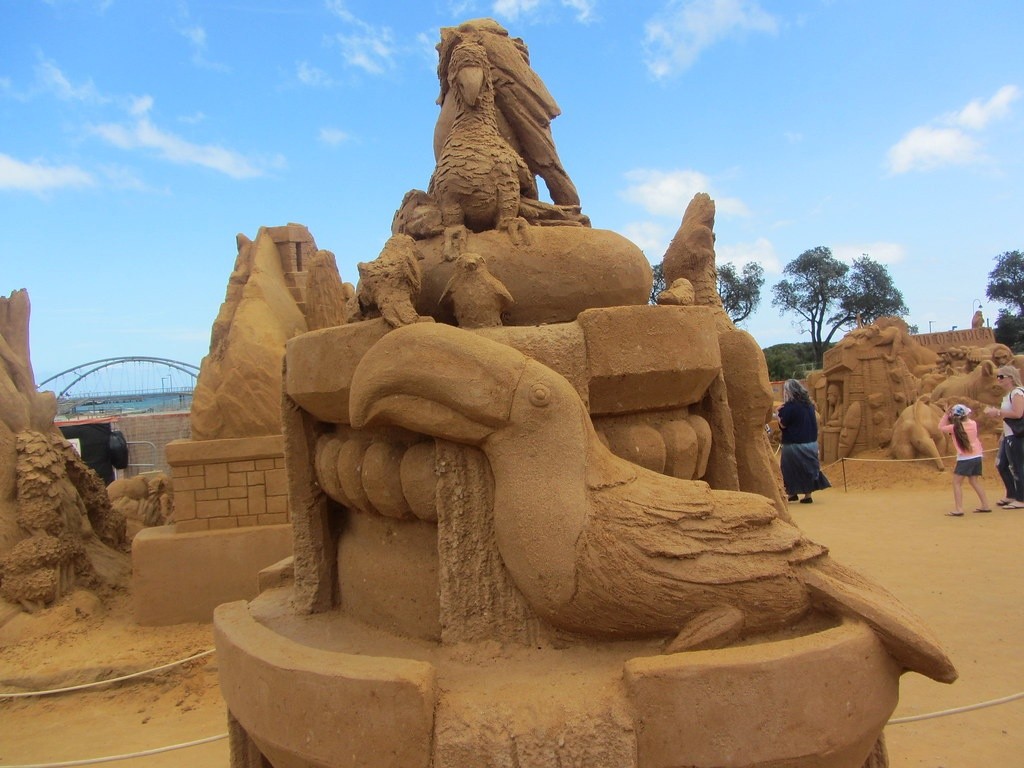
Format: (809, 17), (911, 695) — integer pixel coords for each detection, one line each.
(356, 232), (426, 329)
(436, 252), (517, 329)
(346, 319), (959, 687)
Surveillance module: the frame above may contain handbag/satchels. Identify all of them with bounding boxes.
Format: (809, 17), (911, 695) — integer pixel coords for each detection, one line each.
(1003, 388), (1024, 439)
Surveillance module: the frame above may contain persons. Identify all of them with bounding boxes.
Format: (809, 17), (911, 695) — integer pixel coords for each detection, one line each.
(777, 378), (833, 503)
(937, 401), (992, 517)
(825, 384), (844, 427)
(986, 364), (1024, 510)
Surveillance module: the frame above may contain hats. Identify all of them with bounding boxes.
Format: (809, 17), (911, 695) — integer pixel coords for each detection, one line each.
(950, 404), (971, 418)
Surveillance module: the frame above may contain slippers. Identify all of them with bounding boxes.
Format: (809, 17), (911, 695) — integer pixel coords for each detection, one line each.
(1002, 503), (1024, 509)
(973, 508), (992, 513)
(996, 499), (1012, 506)
(945, 512), (964, 516)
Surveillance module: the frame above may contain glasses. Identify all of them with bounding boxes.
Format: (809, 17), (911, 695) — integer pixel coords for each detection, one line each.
(996, 375), (1012, 380)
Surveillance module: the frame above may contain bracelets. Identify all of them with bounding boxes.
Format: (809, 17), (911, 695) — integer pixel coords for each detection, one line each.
(997, 410), (1001, 416)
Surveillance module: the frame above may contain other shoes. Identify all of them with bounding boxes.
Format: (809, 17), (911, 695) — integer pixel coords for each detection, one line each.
(788, 495), (798, 501)
(800, 498), (813, 504)
